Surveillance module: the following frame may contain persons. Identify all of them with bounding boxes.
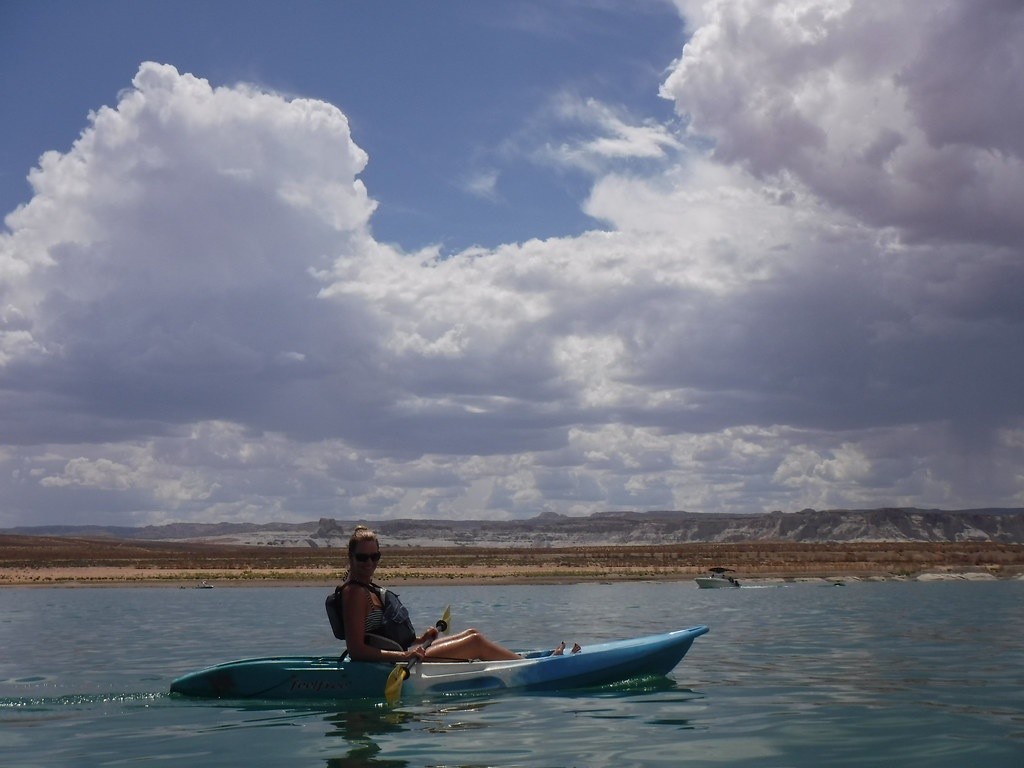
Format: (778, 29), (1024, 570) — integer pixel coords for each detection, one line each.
(325, 526), (582, 662)
(712, 573), (714, 578)
(722, 575), (739, 586)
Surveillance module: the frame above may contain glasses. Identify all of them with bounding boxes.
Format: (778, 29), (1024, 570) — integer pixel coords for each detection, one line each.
(351, 551), (381, 562)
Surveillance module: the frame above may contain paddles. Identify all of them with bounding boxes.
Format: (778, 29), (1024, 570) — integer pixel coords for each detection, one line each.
(385, 602), (451, 704)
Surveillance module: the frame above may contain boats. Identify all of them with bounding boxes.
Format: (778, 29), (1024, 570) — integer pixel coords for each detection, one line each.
(170, 624), (707, 702)
(694, 577), (743, 589)
